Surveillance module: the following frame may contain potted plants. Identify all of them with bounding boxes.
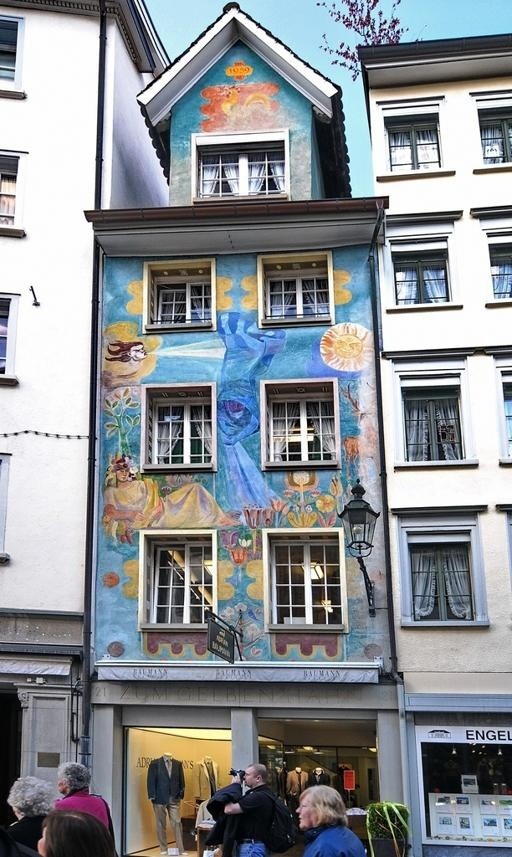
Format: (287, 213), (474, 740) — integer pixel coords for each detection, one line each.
(367, 800), (410, 856)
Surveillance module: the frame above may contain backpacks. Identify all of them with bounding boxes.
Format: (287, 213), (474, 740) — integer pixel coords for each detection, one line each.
(257, 789), (298, 853)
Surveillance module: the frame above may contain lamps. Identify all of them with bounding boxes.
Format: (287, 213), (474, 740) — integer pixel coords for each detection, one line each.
(338, 477), (380, 618)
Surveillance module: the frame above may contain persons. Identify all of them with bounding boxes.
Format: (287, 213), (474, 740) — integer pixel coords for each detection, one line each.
(7, 775), (51, 850)
(102, 457), (147, 525)
(309, 767), (331, 788)
(192, 755), (218, 801)
(285, 766), (308, 832)
(54, 761), (110, 831)
(295, 783), (365, 857)
(37, 810), (116, 857)
(477, 757), (490, 795)
(147, 752), (188, 857)
(223, 763), (275, 856)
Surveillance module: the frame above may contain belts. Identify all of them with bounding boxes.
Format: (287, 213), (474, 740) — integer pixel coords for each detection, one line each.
(238, 839), (260, 843)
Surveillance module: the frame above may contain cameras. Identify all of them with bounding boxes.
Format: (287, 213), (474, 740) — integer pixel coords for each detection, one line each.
(227, 767), (245, 783)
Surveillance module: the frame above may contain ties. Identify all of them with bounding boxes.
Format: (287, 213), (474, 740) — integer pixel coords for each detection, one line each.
(168, 759), (171, 769)
(297, 772), (302, 792)
(317, 774), (320, 784)
